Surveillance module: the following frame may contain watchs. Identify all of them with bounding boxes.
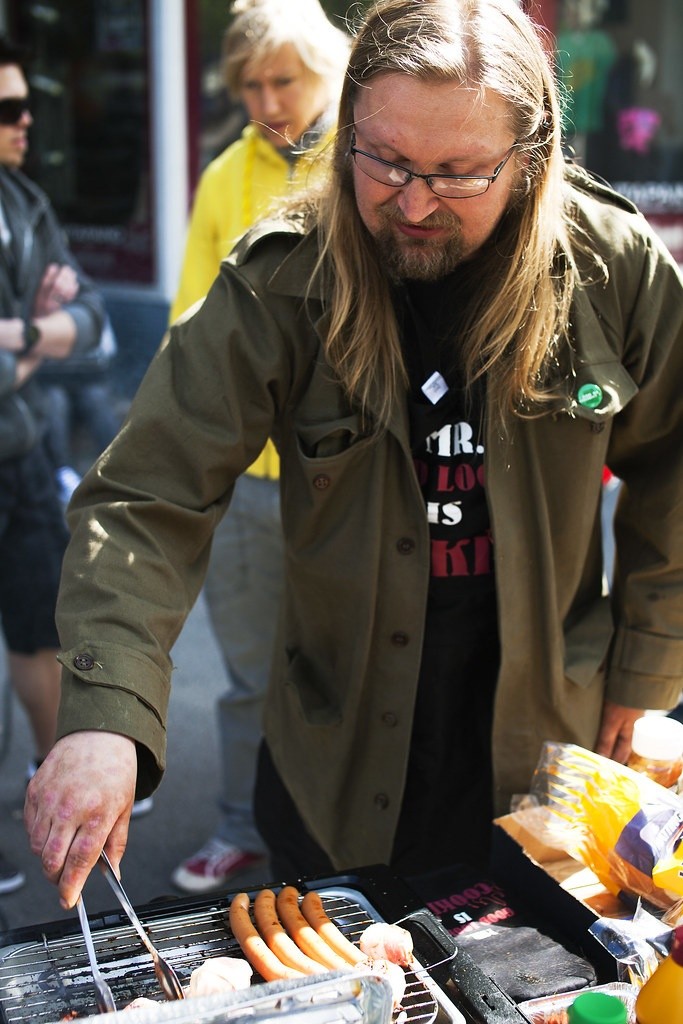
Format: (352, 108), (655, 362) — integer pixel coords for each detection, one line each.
(12, 312), (39, 354)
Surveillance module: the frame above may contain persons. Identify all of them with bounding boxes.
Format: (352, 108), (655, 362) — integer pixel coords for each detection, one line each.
(0, 50), (150, 905)
(20, 1), (683, 908)
(168, 0), (349, 895)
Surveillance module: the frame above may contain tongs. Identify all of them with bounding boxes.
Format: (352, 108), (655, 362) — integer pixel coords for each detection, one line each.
(24, 757), (187, 1015)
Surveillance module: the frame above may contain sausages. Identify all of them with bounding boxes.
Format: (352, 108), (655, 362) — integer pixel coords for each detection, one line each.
(228, 886), (372, 986)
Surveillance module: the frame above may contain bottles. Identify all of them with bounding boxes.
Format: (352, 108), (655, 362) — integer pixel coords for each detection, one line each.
(566, 990), (628, 1024)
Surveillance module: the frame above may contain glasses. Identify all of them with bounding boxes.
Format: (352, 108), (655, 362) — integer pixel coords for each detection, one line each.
(349, 122), (521, 199)
(0, 96), (33, 126)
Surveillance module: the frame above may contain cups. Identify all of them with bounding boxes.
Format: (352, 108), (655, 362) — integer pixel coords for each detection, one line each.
(627, 715), (683, 788)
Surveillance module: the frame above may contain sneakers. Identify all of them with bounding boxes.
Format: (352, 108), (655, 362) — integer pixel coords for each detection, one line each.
(0, 851), (26, 896)
(26, 756), (153, 820)
(170, 836), (258, 895)
